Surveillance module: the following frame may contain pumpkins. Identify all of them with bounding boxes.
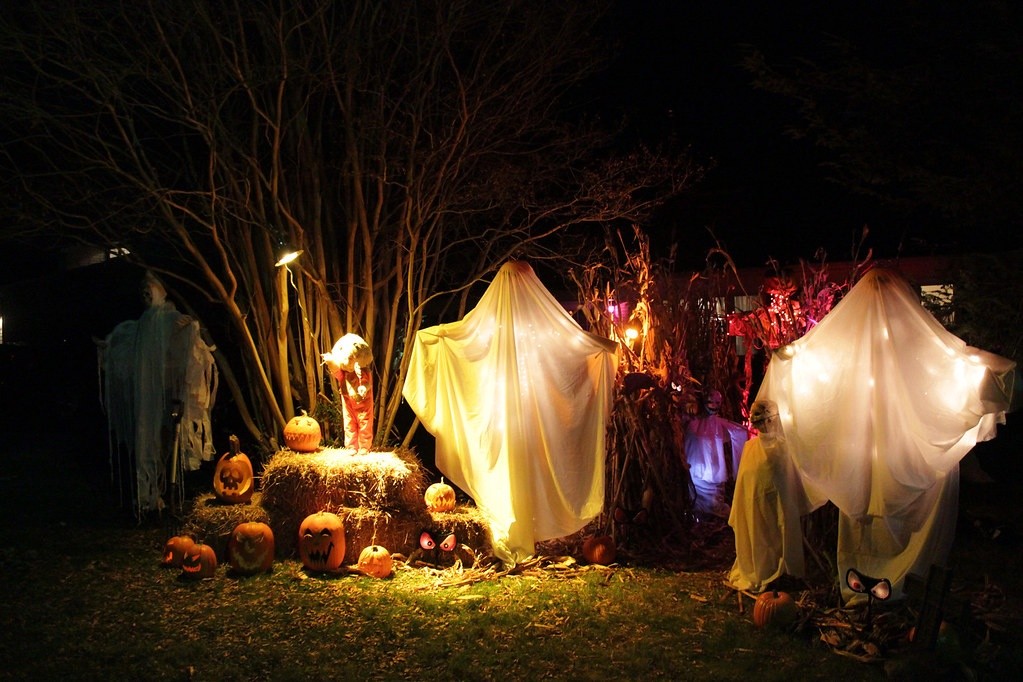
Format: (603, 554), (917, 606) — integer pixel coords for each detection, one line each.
(754, 589), (797, 629)
(582, 529), (616, 564)
